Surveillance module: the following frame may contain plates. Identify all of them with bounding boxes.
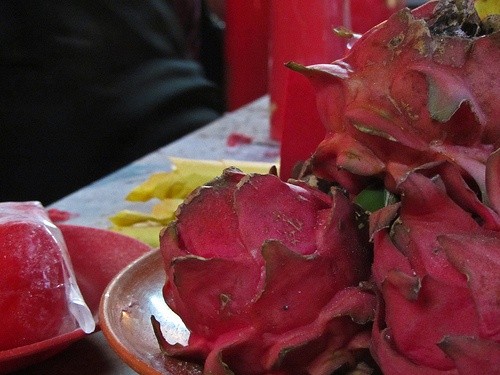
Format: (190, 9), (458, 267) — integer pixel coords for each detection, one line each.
(99, 244), (235, 375)
